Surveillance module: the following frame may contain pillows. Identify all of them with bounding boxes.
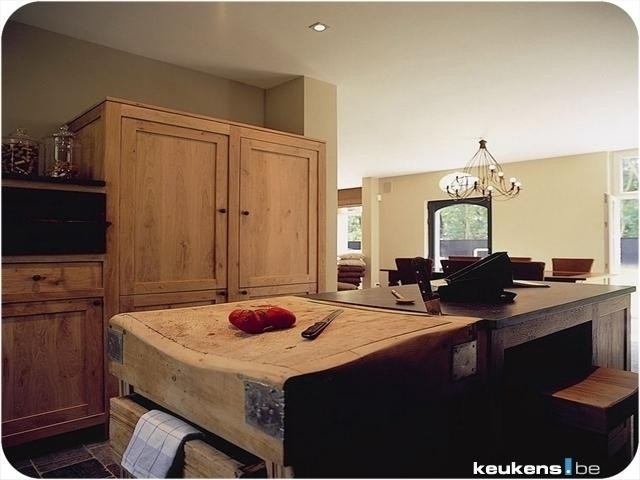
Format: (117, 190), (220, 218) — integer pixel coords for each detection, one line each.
(337, 252), (368, 291)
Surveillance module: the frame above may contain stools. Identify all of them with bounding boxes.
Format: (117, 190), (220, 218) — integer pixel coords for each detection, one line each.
(529, 357), (638, 477)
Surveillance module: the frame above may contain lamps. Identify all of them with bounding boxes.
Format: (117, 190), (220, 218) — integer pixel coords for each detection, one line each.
(445, 138), (523, 203)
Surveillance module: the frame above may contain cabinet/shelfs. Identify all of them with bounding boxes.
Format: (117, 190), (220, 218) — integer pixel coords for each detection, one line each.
(56, 95), (326, 440)
(107, 294), (492, 478)
(2, 174), (107, 450)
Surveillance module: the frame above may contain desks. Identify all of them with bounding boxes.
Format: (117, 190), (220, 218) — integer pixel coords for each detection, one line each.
(305, 275), (636, 459)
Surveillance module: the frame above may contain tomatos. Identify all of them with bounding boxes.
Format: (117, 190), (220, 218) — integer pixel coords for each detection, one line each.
(229, 306), (296, 333)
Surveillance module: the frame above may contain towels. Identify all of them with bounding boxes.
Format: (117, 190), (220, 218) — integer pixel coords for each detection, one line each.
(121, 410), (207, 479)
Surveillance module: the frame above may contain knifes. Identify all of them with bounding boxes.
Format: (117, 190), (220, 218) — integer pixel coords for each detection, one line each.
(411, 256), (442, 317)
(302, 309), (344, 342)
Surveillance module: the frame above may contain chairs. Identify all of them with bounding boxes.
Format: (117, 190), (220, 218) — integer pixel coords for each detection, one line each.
(377, 249), (594, 287)
